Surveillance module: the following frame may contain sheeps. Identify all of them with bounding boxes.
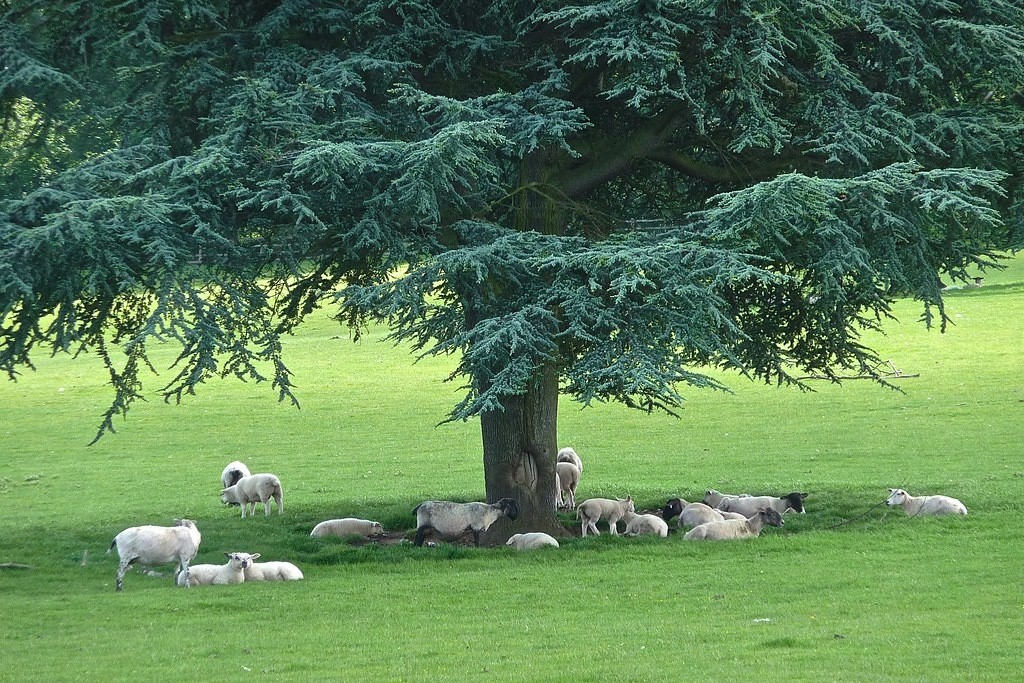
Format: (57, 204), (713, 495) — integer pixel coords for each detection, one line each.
(575, 489), (810, 541)
(218, 460), (284, 519)
(411, 497), (519, 549)
(309, 517), (386, 544)
(555, 446), (584, 512)
(505, 530), (560, 551)
(106, 518), (305, 591)
(885, 488), (968, 521)
